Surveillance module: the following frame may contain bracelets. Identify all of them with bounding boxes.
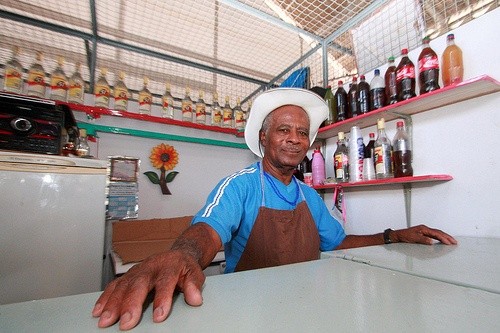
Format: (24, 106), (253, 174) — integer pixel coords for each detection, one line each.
(384, 228), (391, 244)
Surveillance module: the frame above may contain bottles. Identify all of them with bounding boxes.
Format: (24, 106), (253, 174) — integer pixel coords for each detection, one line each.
(28, 51), (46, 98)
(210, 93), (221, 126)
(333, 131), (349, 183)
(63, 129), (90, 158)
(441, 34), (464, 87)
(3, 45), (24, 95)
(181, 87), (193, 122)
(139, 78), (151, 116)
(222, 96), (232, 128)
(233, 97), (244, 129)
(297, 140), (324, 186)
(162, 84), (174, 120)
(68, 62), (84, 106)
(246, 99), (252, 119)
(94, 67), (110, 109)
(113, 72), (128, 112)
(363, 117), (413, 179)
(196, 91), (206, 125)
(417, 37), (440, 95)
(324, 48), (415, 126)
(50, 55), (67, 102)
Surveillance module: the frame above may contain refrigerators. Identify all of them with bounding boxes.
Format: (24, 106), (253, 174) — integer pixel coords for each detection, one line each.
(0, 149), (107, 305)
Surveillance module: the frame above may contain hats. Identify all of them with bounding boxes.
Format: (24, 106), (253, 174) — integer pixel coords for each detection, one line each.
(246, 87), (329, 158)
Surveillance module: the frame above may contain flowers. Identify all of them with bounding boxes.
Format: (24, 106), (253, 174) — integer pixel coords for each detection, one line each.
(142, 144), (180, 195)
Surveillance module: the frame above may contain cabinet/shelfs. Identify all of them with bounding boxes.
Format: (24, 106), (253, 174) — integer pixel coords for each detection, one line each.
(311, 74), (500, 189)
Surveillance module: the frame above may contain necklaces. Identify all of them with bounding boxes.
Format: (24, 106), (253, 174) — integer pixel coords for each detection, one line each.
(257, 161), (299, 206)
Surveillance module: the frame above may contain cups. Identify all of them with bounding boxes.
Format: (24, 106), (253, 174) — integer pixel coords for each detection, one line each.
(303, 172), (312, 186)
(349, 126), (376, 181)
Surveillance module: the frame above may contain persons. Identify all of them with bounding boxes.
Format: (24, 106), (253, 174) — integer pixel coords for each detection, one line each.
(93, 87), (457, 330)
